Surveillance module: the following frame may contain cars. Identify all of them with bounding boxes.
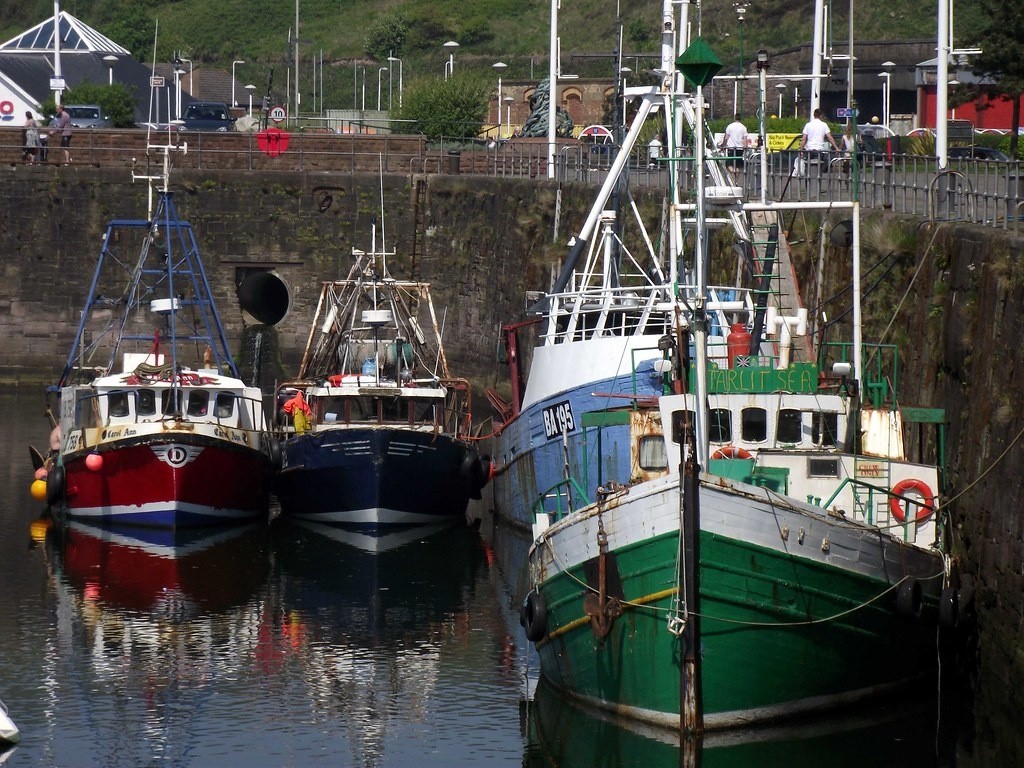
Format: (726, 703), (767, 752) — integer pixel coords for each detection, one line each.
(176, 101), (239, 133)
(48, 103), (115, 133)
(931, 146), (1009, 165)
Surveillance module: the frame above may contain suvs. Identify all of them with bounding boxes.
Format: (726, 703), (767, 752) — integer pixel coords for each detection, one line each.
(762, 133), (889, 169)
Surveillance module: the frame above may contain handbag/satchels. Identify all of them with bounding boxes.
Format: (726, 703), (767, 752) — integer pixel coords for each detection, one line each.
(791, 157), (805, 176)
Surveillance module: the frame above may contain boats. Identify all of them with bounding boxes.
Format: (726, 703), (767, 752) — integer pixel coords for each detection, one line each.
(489, 0), (816, 542)
(276, 219), (485, 532)
(521, 36), (952, 738)
(42, 60), (276, 530)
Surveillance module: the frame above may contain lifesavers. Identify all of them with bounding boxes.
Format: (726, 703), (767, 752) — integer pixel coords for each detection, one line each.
(524, 593), (547, 641)
(889, 479), (935, 526)
(713, 446), (753, 458)
(45, 467), (64, 505)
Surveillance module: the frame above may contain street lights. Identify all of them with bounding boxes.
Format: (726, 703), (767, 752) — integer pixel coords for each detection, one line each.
(104, 40), (789, 136)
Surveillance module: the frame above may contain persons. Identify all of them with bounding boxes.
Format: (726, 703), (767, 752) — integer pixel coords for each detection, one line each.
(838, 118), (864, 191)
(649, 123), (691, 168)
(486, 137), (495, 149)
(800, 109), (840, 193)
(511, 127), (521, 151)
(721, 112), (749, 186)
(21, 105), (71, 166)
